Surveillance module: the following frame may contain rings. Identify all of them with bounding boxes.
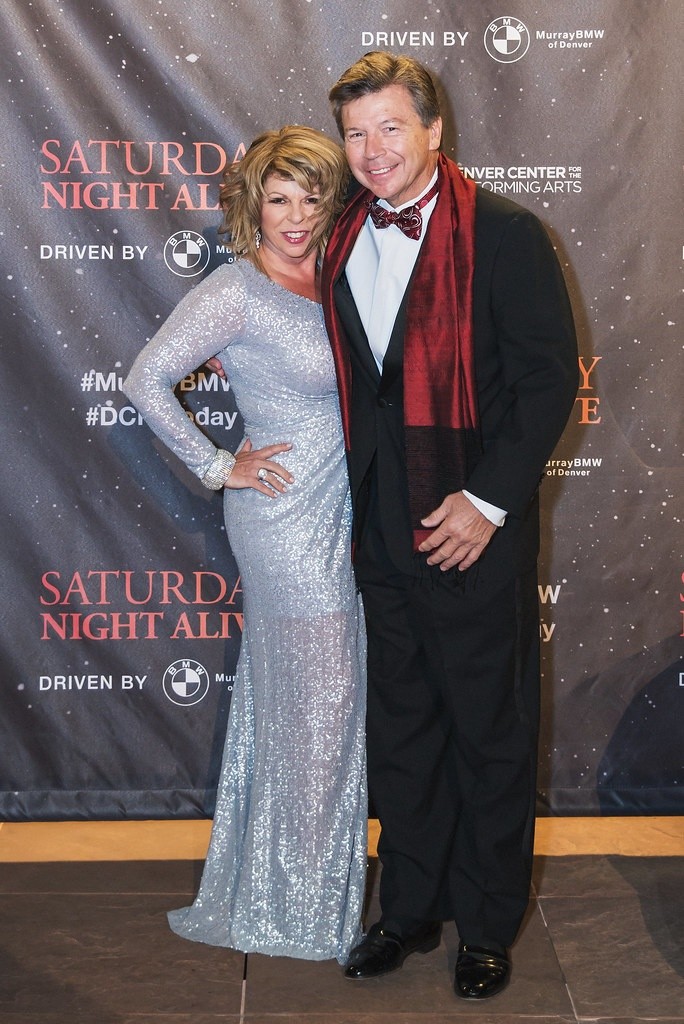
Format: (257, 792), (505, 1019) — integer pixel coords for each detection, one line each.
(257, 468), (268, 479)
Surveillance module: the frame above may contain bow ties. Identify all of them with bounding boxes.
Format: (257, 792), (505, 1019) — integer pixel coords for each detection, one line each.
(363, 178), (440, 241)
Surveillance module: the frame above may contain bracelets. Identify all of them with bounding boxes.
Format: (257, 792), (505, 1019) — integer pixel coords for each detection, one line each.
(200, 449), (236, 490)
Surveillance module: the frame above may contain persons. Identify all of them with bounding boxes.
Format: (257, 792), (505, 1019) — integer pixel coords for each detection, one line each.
(317, 51), (580, 998)
(121, 126), (368, 964)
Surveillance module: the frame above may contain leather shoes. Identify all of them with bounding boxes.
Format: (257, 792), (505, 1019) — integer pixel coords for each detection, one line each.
(454, 939), (513, 1000)
(344, 921), (443, 980)
